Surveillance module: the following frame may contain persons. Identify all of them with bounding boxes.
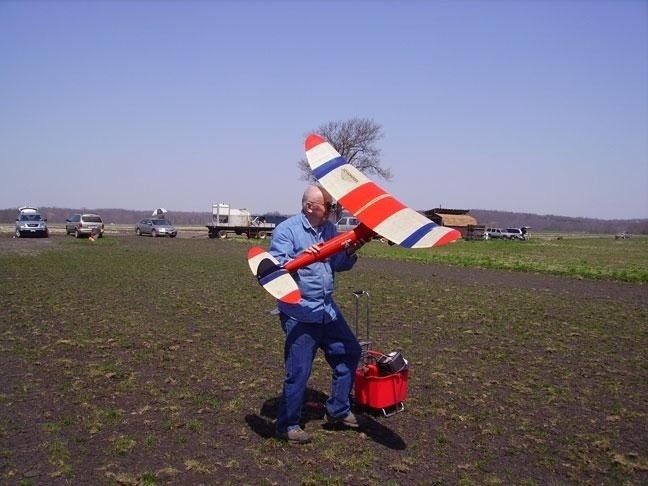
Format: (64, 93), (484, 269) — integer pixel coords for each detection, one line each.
(266, 184), (372, 444)
(521, 225), (528, 241)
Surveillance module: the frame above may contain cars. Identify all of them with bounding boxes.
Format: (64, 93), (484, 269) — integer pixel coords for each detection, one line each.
(135, 218), (177, 238)
(484, 226), (533, 241)
(63, 213), (105, 238)
(331, 216), (362, 233)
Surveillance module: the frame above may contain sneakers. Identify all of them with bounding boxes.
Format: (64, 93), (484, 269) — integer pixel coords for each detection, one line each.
(276, 426), (311, 443)
(324, 411), (361, 427)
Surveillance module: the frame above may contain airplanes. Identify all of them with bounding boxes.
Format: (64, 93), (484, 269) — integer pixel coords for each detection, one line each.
(243, 132), (463, 307)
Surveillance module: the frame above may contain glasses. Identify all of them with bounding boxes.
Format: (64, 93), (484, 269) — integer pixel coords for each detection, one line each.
(310, 202), (336, 210)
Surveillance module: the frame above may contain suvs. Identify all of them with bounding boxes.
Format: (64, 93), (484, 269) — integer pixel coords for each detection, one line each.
(14, 205), (49, 236)
